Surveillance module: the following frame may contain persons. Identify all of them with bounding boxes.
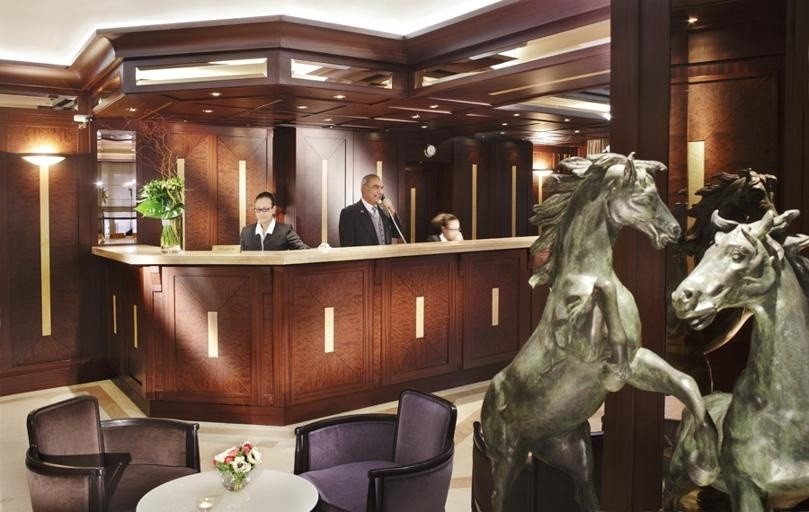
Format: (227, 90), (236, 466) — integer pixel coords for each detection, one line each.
(425, 212), (465, 242)
(239, 190), (312, 251)
(338, 175), (405, 246)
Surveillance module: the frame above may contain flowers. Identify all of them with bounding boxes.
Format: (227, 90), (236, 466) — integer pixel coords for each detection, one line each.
(211, 441), (264, 490)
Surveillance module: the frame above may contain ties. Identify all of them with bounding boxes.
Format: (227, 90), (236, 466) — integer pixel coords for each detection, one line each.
(372, 207), (378, 223)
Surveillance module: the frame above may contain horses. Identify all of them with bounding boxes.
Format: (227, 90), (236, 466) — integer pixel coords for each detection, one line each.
(672, 168), (779, 395)
(671, 208), (809, 512)
(480, 150), (707, 511)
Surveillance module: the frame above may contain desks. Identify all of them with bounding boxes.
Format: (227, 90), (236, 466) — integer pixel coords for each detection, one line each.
(136, 469), (319, 512)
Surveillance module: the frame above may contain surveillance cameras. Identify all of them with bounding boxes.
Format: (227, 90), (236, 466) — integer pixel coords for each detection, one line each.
(74, 114), (93, 127)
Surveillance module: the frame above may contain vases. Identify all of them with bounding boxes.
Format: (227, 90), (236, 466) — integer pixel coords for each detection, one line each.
(220, 469), (252, 492)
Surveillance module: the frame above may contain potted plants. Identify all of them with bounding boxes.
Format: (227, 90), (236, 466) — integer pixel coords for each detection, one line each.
(133, 176), (186, 256)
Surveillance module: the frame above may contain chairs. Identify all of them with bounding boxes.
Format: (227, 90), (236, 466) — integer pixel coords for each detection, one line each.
(25, 395), (210, 512)
(294, 388), (457, 512)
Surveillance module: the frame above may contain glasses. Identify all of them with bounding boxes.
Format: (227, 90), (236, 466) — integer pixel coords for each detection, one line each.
(253, 205), (274, 213)
(445, 227), (462, 233)
